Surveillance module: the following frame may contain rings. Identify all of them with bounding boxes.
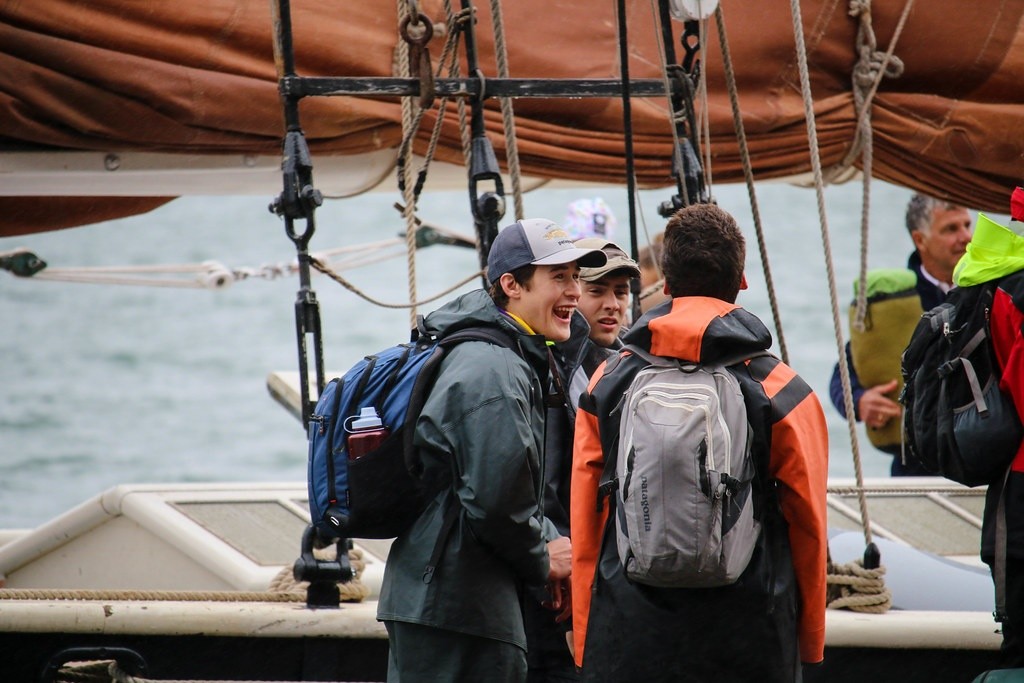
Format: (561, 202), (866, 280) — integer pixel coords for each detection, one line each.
(879, 415), (884, 421)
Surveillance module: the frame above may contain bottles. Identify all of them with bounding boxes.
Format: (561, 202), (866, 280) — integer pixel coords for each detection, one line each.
(344, 406), (387, 459)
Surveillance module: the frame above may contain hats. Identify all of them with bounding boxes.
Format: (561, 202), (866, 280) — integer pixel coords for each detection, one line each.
(573, 238), (641, 282)
(486, 218), (608, 285)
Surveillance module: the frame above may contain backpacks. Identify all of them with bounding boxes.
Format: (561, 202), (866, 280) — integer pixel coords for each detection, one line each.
(614, 342), (779, 589)
(900, 279), (1024, 488)
(308, 314), (529, 539)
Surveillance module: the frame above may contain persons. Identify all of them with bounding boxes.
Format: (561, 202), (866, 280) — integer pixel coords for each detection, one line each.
(829, 185), (972, 477)
(377, 214), (610, 683)
(639, 232), (673, 292)
(565, 202), (829, 683)
(952, 175), (1024, 683)
(567, 235), (635, 436)
